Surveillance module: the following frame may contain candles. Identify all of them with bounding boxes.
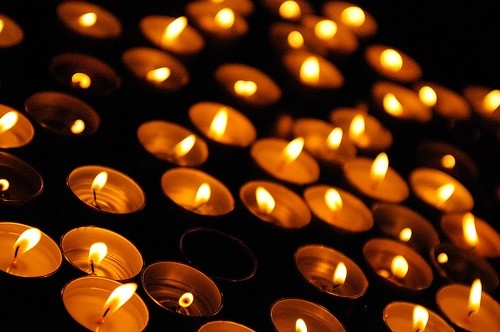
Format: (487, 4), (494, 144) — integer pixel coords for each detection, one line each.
(0, 0), (500, 332)
